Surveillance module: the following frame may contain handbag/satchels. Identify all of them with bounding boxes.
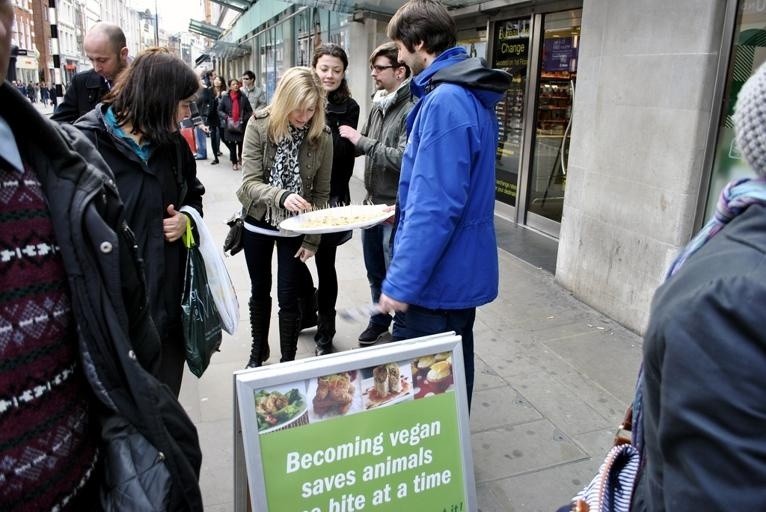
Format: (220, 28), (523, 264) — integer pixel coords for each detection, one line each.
(557, 444), (640, 512)
(179, 205), (238, 378)
(224, 210), (243, 256)
(227, 117), (243, 134)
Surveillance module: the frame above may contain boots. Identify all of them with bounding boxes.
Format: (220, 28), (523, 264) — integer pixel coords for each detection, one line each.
(246, 289), (336, 368)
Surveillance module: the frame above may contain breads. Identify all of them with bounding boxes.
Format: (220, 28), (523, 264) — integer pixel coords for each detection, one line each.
(312, 372), (354, 416)
(412, 352), (453, 381)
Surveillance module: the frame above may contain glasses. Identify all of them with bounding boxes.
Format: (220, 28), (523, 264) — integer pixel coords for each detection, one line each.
(370, 65), (393, 73)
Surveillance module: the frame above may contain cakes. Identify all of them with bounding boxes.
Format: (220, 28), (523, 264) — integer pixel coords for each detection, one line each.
(372, 365), (402, 398)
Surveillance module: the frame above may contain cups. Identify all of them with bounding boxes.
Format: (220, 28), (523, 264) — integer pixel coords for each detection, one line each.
(425, 375), (453, 395)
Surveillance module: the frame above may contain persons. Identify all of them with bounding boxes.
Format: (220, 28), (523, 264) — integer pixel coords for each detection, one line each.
(71, 44), (205, 398)
(231, 66), (336, 370)
(293, 42), (361, 356)
(336, 43), (421, 346)
(376, 1), (516, 422)
(0, 0), (203, 512)
(630, 61), (766, 512)
(2, 68), (267, 170)
(47, 20), (139, 122)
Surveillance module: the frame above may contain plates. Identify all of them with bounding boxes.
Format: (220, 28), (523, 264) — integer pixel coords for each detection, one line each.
(363, 378), (412, 408)
(258, 392), (309, 434)
(279, 204), (395, 235)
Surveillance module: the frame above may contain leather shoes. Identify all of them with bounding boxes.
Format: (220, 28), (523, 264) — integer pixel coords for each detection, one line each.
(358, 324), (388, 344)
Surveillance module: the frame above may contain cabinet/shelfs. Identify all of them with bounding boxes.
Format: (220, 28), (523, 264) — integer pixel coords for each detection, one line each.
(494, 76), (576, 206)
